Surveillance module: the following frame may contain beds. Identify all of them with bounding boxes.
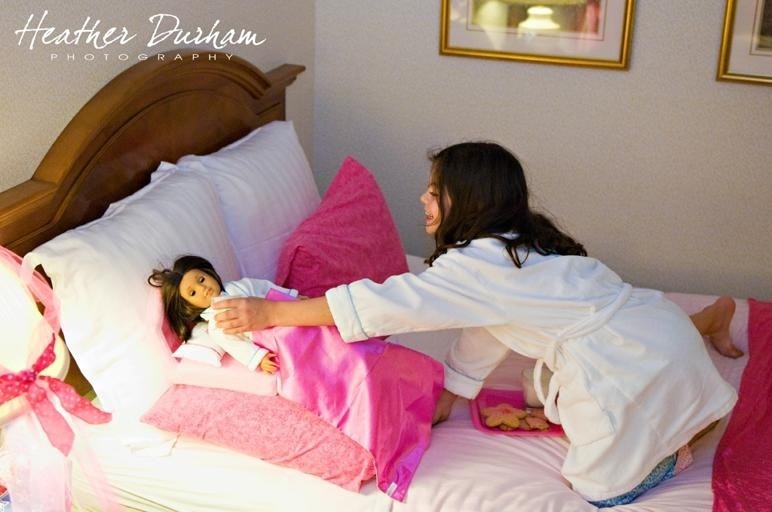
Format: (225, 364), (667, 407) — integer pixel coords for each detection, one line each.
(0, 49), (771, 511)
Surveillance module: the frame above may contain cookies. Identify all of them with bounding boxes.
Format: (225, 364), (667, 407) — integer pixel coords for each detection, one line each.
(480, 403), (550, 431)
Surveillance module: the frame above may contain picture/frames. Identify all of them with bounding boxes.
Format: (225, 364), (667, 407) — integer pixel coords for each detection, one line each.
(440, 0), (636, 70)
(716, 0), (772, 86)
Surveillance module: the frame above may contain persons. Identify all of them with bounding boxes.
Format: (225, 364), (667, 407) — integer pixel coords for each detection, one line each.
(147, 255), (446, 505)
(210, 140), (744, 507)
